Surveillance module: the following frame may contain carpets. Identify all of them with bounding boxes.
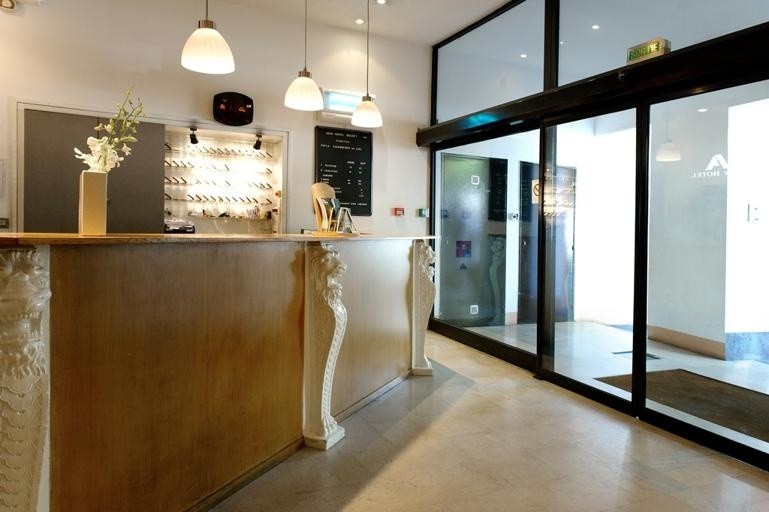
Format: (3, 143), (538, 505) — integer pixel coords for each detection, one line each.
(593, 368), (768, 444)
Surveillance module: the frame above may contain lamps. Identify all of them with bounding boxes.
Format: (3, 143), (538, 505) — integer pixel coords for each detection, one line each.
(654, 114), (682, 162)
(284, 0), (324, 111)
(181, 0), (235, 74)
(189, 127), (197, 143)
(253, 133), (263, 150)
(350, 0), (382, 128)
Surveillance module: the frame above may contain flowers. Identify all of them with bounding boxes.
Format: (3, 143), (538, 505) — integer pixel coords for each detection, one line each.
(73, 87), (144, 174)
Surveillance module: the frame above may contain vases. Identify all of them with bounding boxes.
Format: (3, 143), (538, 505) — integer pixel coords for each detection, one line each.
(78, 170), (108, 237)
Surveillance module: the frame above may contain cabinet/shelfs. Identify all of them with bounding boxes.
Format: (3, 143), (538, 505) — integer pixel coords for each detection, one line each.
(163, 125), (289, 237)
